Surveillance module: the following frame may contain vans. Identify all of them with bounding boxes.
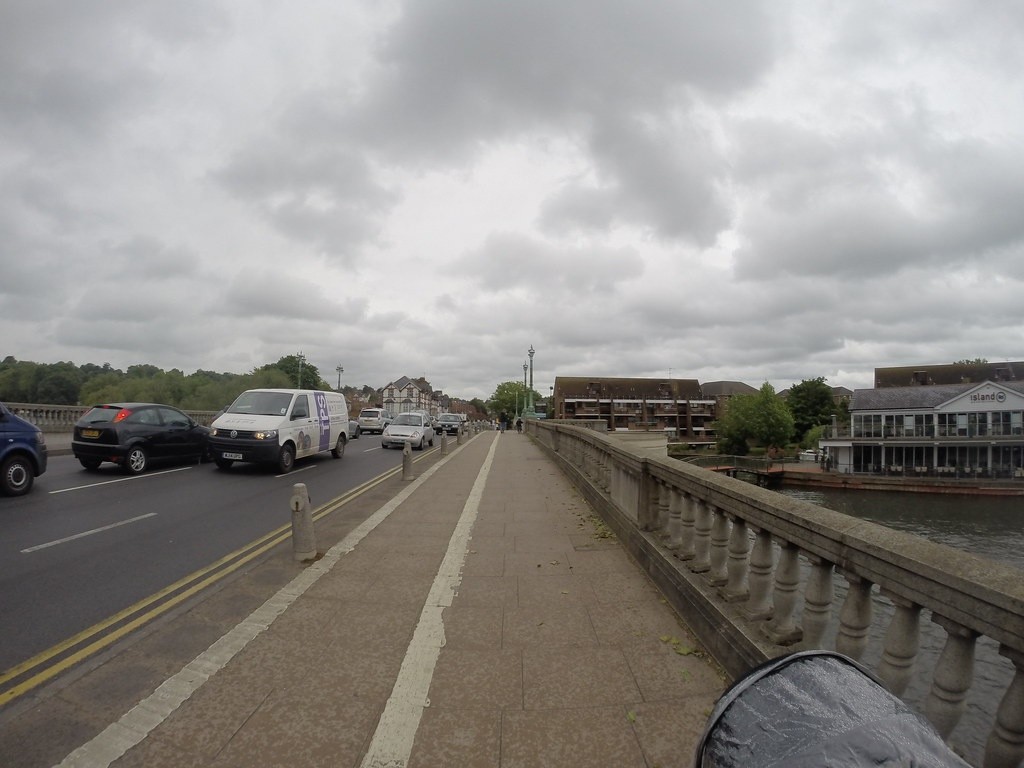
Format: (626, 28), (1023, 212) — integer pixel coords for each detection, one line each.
(207, 389), (350, 474)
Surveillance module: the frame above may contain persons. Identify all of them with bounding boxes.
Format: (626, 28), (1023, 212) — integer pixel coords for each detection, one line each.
(821, 452), (828, 467)
(515, 417), (524, 434)
(498, 408), (508, 433)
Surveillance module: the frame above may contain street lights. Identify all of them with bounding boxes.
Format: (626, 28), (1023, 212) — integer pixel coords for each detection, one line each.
(523, 361), (528, 412)
(528, 344), (536, 415)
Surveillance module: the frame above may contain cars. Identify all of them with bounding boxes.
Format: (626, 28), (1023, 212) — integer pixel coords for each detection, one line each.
(71, 402), (214, 475)
(0, 401), (48, 496)
(436, 414), (466, 435)
(410, 410), (438, 430)
(349, 421), (360, 439)
(381, 412), (434, 450)
(358, 408), (393, 434)
